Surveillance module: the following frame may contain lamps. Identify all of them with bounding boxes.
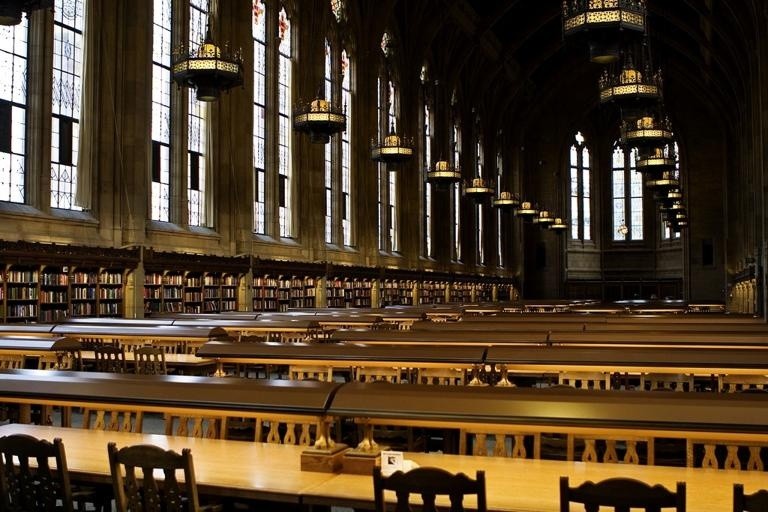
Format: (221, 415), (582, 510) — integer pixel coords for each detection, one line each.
(614, 110), (689, 234)
(562, 1), (648, 47)
(170, 1), (244, 101)
(422, 83), (462, 190)
(293, 2), (346, 146)
(464, 133), (568, 230)
(597, 41), (663, 104)
(370, 79), (414, 172)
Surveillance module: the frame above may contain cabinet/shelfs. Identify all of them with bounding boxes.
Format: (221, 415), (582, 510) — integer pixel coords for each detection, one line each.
(0, 232), (523, 322)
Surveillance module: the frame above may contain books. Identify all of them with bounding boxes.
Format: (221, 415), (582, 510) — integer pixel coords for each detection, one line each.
(253, 275), (316, 312)
(326, 280), (372, 308)
(2, 271), (122, 322)
(380, 279), (517, 306)
(143, 271), (238, 314)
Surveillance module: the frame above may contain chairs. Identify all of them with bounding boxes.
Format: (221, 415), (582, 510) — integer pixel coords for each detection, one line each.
(0, 297), (768, 512)
